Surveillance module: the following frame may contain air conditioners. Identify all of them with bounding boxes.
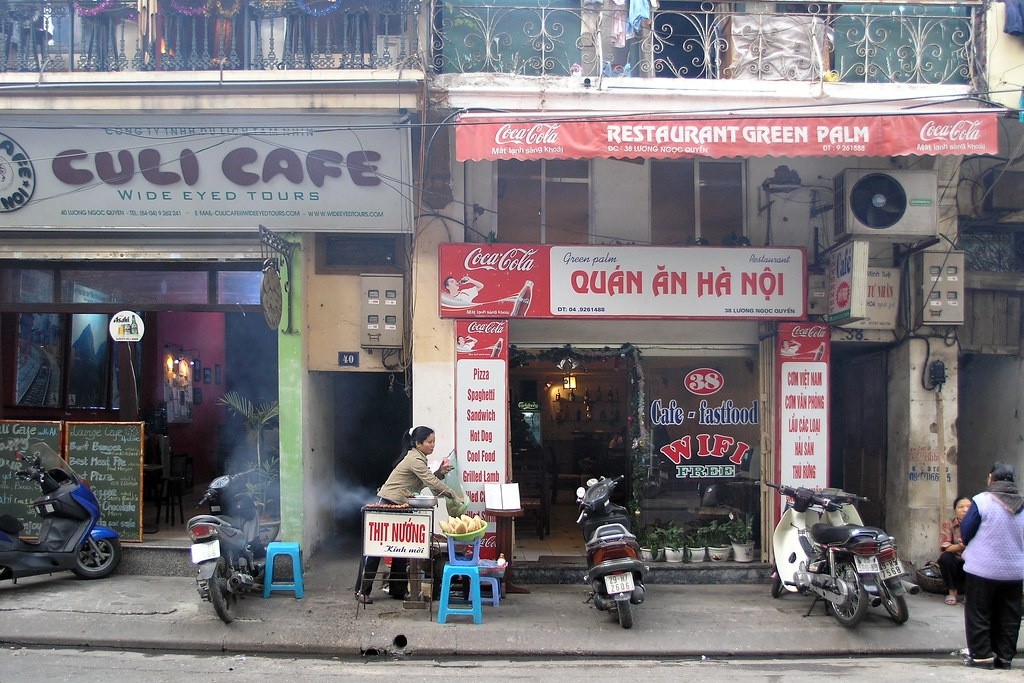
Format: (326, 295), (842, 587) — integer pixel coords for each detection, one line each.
(832, 165), (941, 245)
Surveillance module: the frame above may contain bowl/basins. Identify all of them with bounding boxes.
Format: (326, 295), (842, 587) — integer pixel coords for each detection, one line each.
(443, 520), (488, 541)
(407, 496), (439, 507)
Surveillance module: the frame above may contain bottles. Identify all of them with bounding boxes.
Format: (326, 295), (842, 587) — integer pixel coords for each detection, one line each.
(131, 315), (138, 334)
(490, 337), (504, 358)
(497, 553), (507, 567)
(510, 280), (534, 317)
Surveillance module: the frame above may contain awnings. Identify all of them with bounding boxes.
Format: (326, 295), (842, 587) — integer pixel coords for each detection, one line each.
(450, 100), (1010, 165)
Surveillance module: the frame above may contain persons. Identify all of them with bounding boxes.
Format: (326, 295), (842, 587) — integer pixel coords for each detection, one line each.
(958, 460), (1024, 670)
(939, 495), (979, 606)
(354, 424), (464, 604)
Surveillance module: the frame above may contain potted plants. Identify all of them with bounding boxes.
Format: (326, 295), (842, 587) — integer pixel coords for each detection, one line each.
(640, 514), (756, 563)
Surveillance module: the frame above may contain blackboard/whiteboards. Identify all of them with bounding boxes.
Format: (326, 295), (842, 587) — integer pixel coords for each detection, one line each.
(65, 421), (144, 542)
(0, 420), (62, 540)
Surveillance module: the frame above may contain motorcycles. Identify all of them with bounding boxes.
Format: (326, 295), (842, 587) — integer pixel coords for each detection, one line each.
(575, 474), (646, 628)
(186, 468), (267, 626)
(765, 481), (920, 629)
(0, 441), (121, 587)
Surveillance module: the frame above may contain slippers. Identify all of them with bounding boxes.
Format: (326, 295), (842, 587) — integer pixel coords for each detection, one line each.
(944, 595), (957, 605)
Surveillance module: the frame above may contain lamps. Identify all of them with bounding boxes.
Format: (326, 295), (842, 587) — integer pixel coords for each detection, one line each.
(560, 360), (578, 392)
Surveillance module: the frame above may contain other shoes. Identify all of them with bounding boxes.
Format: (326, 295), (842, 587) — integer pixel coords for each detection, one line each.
(393, 593), (409, 600)
(963, 650), (998, 670)
(355, 592), (372, 604)
(994, 657), (1011, 670)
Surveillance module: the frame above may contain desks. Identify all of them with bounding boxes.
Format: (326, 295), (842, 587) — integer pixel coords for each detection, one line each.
(512, 459), (552, 542)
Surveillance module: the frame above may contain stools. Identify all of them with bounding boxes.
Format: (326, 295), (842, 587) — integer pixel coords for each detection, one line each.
(446, 533), (481, 567)
(263, 541), (307, 599)
(471, 575), (503, 607)
(436, 562), (482, 625)
